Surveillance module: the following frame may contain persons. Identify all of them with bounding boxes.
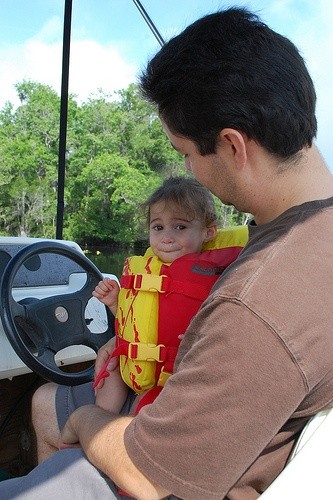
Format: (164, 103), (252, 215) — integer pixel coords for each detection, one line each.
(30, 4), (333, 499)
(92, 175), (228, 417)
